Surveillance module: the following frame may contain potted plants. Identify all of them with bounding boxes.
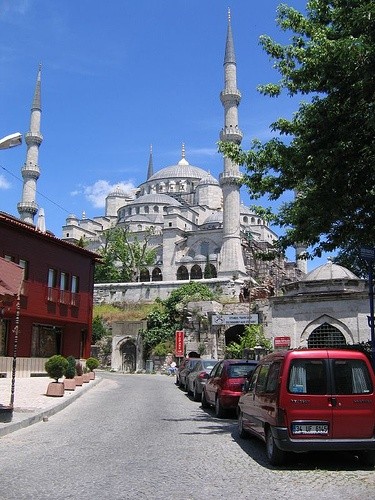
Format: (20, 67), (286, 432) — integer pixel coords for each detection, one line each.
(83, 366), (90, 383)
(86, 357), (99, 379)
(63, 356), (76, 390)
(45, 355), (69, 396)
(74, 361), (83, 385)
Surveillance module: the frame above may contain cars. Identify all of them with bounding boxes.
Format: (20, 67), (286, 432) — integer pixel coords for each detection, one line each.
(176, 357), (260, 417)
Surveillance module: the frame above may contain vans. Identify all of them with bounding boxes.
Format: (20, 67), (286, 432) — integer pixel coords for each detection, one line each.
(235, 348), (375, 466)
